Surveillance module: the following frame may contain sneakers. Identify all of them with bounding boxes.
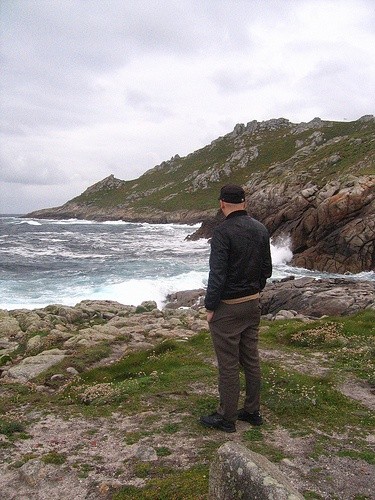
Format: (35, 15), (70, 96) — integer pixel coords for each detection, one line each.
(236, 408), (263, 426)
(200, 412), (236, 433)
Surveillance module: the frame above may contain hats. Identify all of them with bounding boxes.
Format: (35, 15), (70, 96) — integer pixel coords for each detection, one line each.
(217, 184), (245, 204)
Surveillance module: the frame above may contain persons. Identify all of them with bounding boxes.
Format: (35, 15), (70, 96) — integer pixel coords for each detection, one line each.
(201, 184), (272, 432)
